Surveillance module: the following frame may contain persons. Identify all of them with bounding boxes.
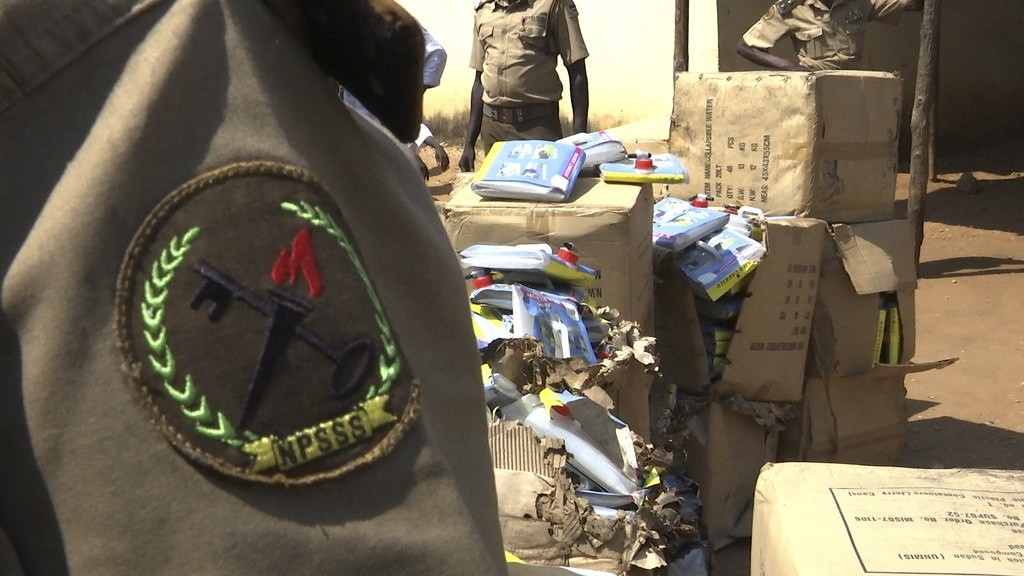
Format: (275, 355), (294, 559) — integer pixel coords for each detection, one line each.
(0, 0), (508, 576)
(736, 0), (926, 71)
(457, 0), (589, 174)
(404, 22), (450, 182)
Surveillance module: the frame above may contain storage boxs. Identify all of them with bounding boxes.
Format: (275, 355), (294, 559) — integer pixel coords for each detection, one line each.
(444, 67), (1024, 576)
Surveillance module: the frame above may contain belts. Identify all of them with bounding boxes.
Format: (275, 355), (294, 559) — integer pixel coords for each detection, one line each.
(482, 101), (559, 125)
